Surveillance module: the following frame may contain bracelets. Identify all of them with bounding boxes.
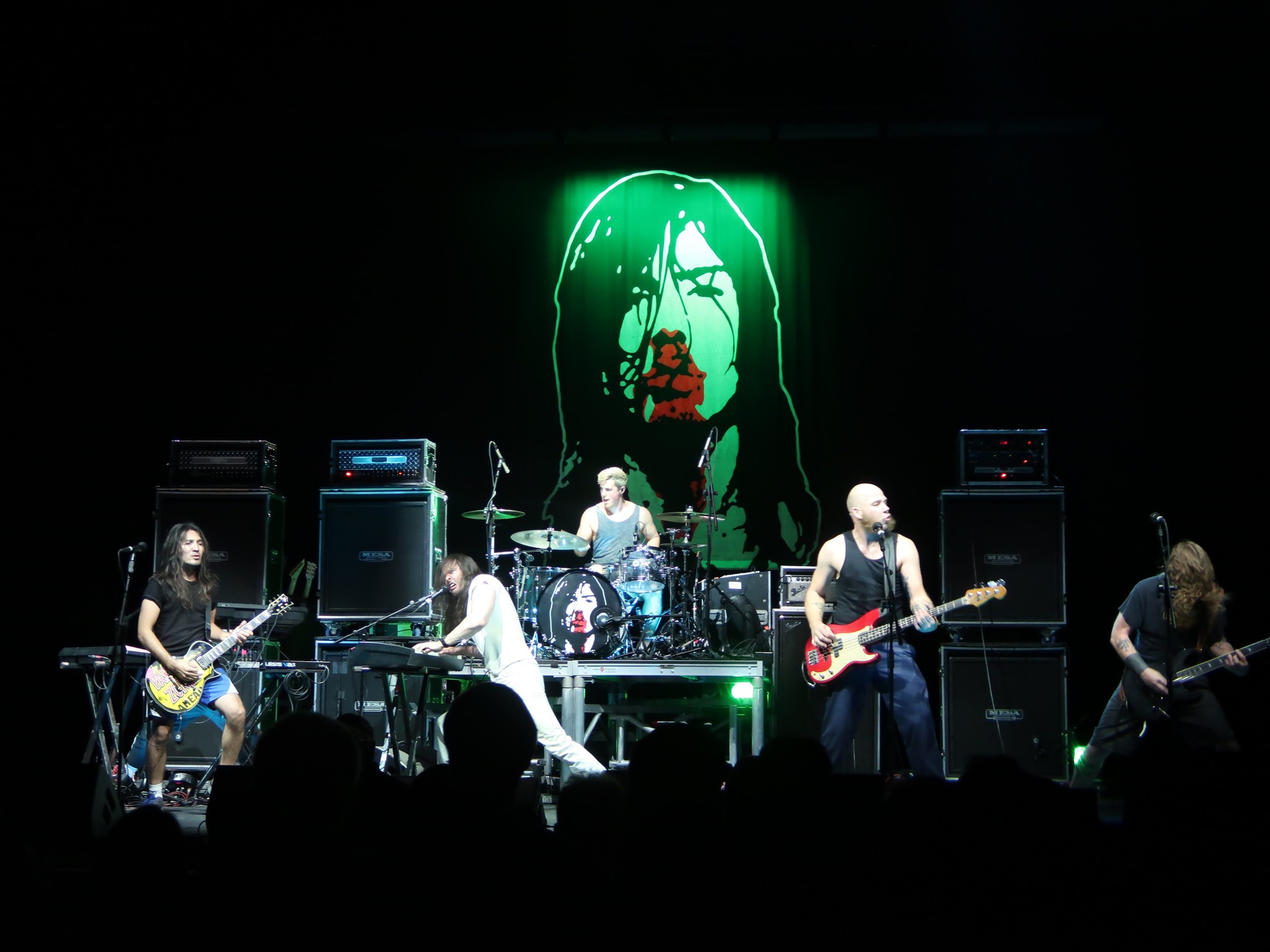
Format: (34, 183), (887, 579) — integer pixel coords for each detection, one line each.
(1125, 653), (1149, 675)
(440, 637), (449, 650)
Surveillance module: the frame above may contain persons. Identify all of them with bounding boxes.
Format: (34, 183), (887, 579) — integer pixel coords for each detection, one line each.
(553, 574), (606, 652)
(0, 668), (1270, 952)
(573, 466), (665, 650)
(412, 553), (606, 776)
(804, 483), (946, 782)
(112, 523), (246, 816)
(1069, 540), (1245, 795)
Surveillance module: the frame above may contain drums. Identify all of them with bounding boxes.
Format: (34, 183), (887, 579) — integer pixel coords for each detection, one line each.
(616, 547), (667, 579)
(536, 569), (629, 658)
(512, 566), (571, 622)
(615, 581), (665, 650)
(602, 564), (650, 582)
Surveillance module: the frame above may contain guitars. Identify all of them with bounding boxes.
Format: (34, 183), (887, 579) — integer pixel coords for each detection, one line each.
(146, 593), (294, 715)
(804, 578), (1008, 684)
(1123, 637), (1270, 725)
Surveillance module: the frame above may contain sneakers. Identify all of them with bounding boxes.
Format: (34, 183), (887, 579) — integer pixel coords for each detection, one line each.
(143, 792), (164, 806)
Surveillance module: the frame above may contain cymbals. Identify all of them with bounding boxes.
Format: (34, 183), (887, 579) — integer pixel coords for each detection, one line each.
(461, 509), (525, 520)
(660, 542), (708, 549)
(655, 511), (725, 523)
(510, 529), (589, 550)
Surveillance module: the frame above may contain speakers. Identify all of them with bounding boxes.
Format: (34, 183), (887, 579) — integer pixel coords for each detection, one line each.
(206, 765), (248, 832)
(79, 763), (123, 840)
(319, 491), (448, 620)
(779, 615), (874, 772)
(166, 670), (256, 761)
(940, 493), (1066, 626)
(154, 490), (288, 608)
(946, 656), (1065, 781)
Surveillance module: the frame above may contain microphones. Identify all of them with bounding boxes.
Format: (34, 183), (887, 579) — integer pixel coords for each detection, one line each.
(872, 522), (886, 540)
(744, 610), (758, 624)
(121, 541), (148, 553)
(1149, 512), (1166, 523)
(174, 730), (183, 742)
(493, 442), (510, 473)
(597, 613), (622, 628)
(698, 428), (714, 468)
(523, 552), (534, 561)
(418, 584), (451, 609)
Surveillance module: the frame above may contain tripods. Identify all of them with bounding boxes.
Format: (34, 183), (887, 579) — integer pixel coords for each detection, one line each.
(608, 450), (744, 661)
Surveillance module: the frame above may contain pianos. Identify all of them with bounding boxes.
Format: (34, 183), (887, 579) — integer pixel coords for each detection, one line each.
(59, 643), (330, 805)
(347, 640), (466, 779)
